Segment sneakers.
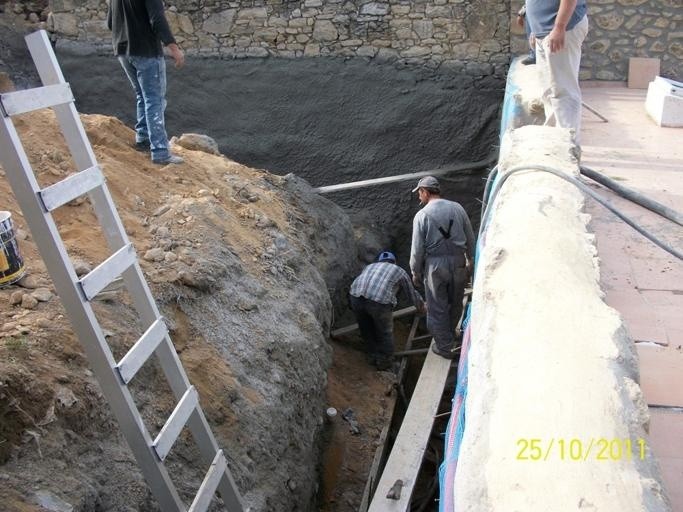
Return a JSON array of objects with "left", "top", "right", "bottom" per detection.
[
  {"left": 522, "top": 58, "right": 535, "bottom": 64},
  {"left": 369, "top": 359, "right": 392, "bottom": 370},
  {"left": 136, "top": 140, "right": 183, "bottom": 163},
  {"left": 432, "top": 344, "right": 453, "bottom": 358}
]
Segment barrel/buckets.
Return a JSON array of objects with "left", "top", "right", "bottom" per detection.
[{"left": 0, "top": 210, "right": 27, "bottom": 289}]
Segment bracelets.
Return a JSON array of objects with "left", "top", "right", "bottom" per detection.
[{"left": 517, "top": 12, "right": 525, "bottom": 18}]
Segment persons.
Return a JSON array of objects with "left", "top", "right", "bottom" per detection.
[
  {"left": 516, "top": 0, "right": 536, "bottom": 67},
  {"left": 349, "top": 250, "right": 427, "bottom": 372},
  {"left": 106, "top": 0, "right": 186, "bottom": 166},
  {"left": 525, "top": 0, "right": 589, "bottom": 145},
  {"left": 407, "top": 176, "right": 477, "bottom": 359}
]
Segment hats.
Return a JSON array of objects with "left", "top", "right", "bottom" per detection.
[
  {"left": 379, "top": 252, "right": 395, "bottom": 262},
  {"left": 412, "top": 176, "right": 440, "bottom": 192}
]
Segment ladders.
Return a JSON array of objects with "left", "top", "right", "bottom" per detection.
[{"left": 0, "top": 28, "right": 247, "bottom": 512}]
